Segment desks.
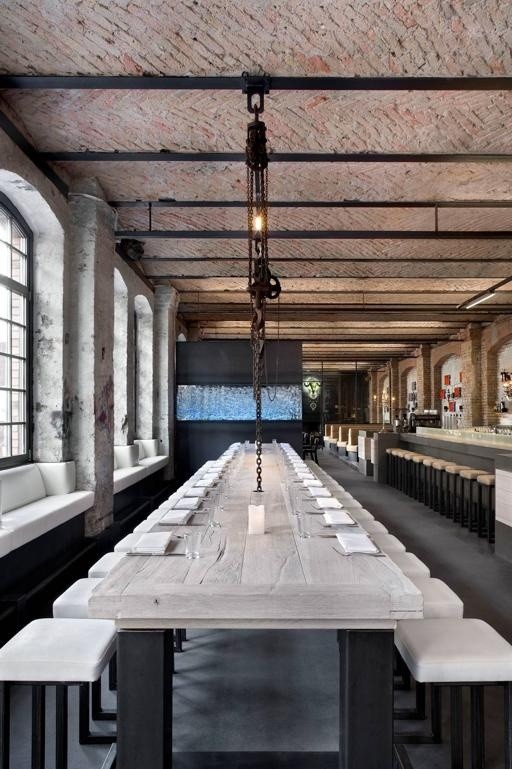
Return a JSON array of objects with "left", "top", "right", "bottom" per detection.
[{"left": 88, "top": 443, "right": 424, "bottom": 769}]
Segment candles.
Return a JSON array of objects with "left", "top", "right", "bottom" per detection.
[{"left": 249, "top": 504, "right": 266, "bottom": 536}]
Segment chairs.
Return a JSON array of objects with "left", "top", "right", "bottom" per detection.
[{"left": 301, "top": 432, "right": 320, "bottom": 466}]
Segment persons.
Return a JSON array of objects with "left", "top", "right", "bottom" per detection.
[{"left": 393, "top": 407, "right": 416, "bottom": 432}]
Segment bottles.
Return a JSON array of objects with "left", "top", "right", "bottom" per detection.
[
  {"left": 494, "top": 397, "right": 505, "bottom": 412},
  {"left": 500, "top": 367, "right": 506, "bottom": 382}
]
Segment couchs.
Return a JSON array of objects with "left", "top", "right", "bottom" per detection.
[{"left": 0, "top": 463, "right": 97, "bottom": 618}]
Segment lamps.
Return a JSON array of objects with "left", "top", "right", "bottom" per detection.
[{"left": 233, "top": 76, "right": 282, "bottom": 492}]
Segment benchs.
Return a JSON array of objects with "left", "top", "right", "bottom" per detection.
[
  {"left": 323, "top": 423, "right": 392, "bottom": 461},
  {"left": 112, "top": 439, "right": 169, "bottom": 515}
]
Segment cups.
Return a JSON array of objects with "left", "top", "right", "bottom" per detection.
[
  {"left": 272, "top": 439, "right": 312, "bottom": 539},
  {"left": 183, "top": 440, "right": 250, "bottom": 560}
]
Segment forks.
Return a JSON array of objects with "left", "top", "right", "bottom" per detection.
[{"left": 332, "top": 546, "right": 386, "bottom": 558}]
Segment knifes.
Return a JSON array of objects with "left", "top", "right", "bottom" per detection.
[
  {"left": 159, "top": 523, "right": 204, "bottom": 527},
  {"left": 320, "top": 534, "right": 372, "bottom": 540}
]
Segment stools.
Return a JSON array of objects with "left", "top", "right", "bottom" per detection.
[
  {"left": 386, "top": 446, "right": 495, "bottom": 546},
  {"left": 392, "top": 617, "right": 510, "bottom": 769},
  {"left": 54, "top": 460, "right": 217, "bottom": 742},
  {"left": 2, "top": 618, "right": 117, "bottom": 769},
  {"left": 303, "top": 460, "right": 464, "bottom": 744}
]
